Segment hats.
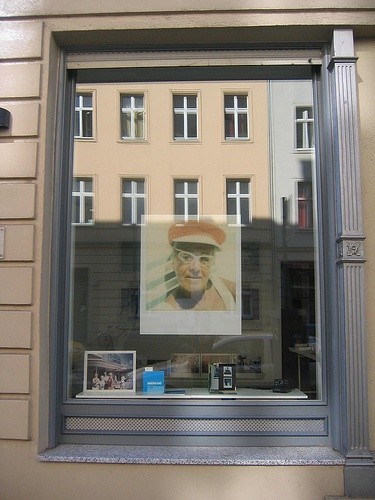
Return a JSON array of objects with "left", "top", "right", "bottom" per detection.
[{"left": 169, "top": 221, "right": 225, "bottom": 250}]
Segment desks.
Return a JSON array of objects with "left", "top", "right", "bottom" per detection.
[{"left": 75, "top": 386, "right": 309, "bottom": 400}]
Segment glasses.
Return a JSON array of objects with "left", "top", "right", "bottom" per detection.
[{"left": 173, "top": 247, "right": 216, "bottom": 268}]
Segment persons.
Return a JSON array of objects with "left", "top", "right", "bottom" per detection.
[
  {"left": 145, "top": 219, "right": 237, "bottom": 310},
  {"left": 91, "top": 371, "right": 127, "bottom": 390}
]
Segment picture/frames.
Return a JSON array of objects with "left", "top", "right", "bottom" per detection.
[
  {"left": 82, "top": 350, "right": 137, "bottom": 394},
  {"left": 219, "top": 363, "right": 237, "bottom": 391}
]
